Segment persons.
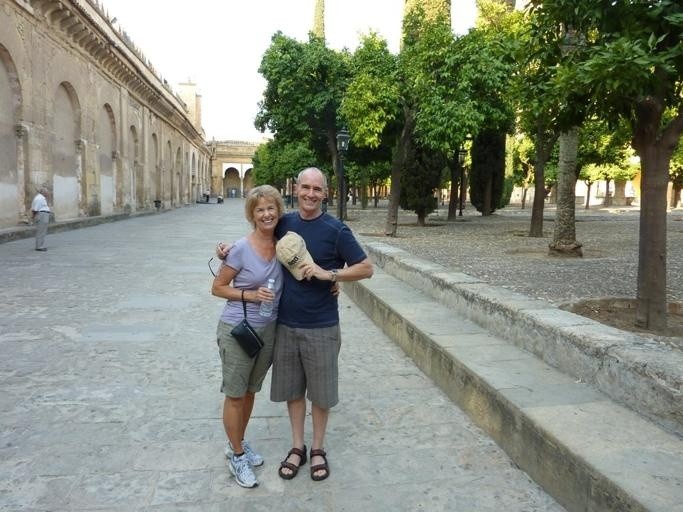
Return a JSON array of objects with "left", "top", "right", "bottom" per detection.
[
  {"left": 215, "top": 167, "right": 373, "bottom": 480},
  {"left": 204, "top": 188, "right": 210, "bottom": 201},
  {"left": 30, "top": 187, "right": 50, "bottom": 251},
  {"left": 210, "top": 185, "right": 341, "bottom": 489}
]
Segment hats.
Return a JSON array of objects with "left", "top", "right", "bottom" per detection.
[{"left": 275, "top": 230, "right": 314, "bottom": 281}]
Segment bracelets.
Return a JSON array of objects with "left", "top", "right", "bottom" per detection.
[{"left": 328, "top": 268, "right": 336, "bottom": 283}]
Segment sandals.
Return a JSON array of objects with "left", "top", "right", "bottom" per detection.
[
  {"left": 278, "top": 445, "right": 307, "bottom": 479},
  {"left": 310, "top": 447, "right": 330, "bottom": 481}
]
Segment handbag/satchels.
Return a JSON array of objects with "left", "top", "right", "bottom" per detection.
[{"left": 230, "top": 289, "right": 264, "bottom": 358}]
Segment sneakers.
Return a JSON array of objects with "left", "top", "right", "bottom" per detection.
[
  {"left": 228, "top": 453, "right": 259, "bottom": 488},
  {"left": 224, "top": 440, "right": 264, "bottom": 466}
]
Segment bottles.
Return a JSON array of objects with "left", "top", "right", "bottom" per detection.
[{"left": 258, "top": 278, "right": 276, "bottom": 317}]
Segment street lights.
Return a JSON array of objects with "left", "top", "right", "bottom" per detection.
[
  {"left": 457, "top": 146, "right": 465, "bottom": 215},
  {"left": 336, "top": 124, "right": 349, "bottom": 222},
  {"left": 372, "top": 175, "right": 376, "bottom": 207}
]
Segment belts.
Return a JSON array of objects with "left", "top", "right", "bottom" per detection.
[{"left": 41, "top": 211, "right": 49, "bottom": 213}]
